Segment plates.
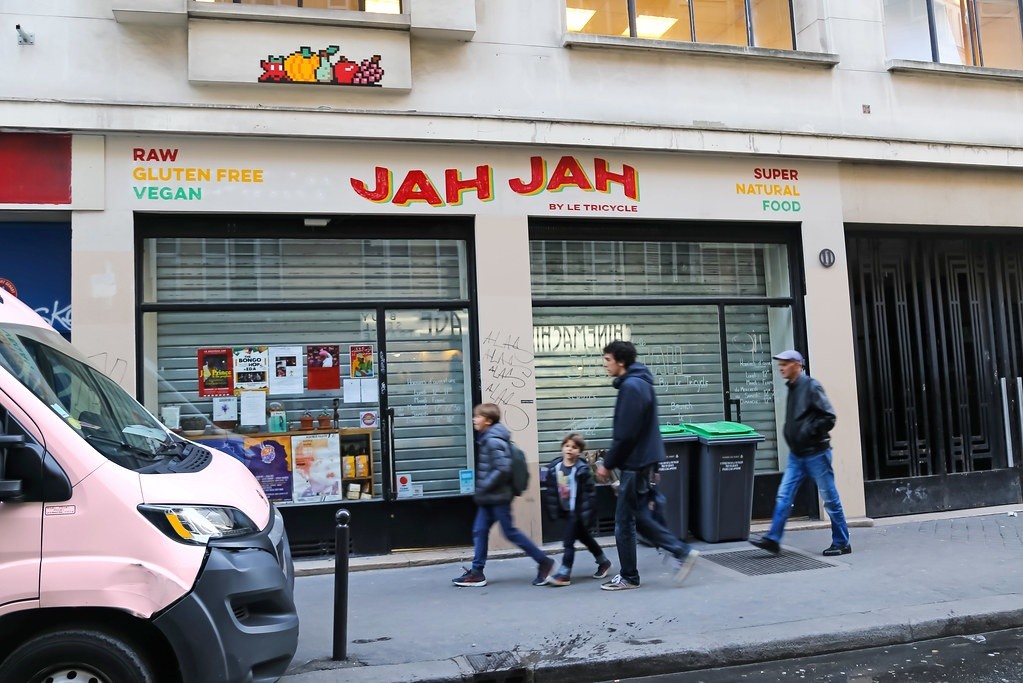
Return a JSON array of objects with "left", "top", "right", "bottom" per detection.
[
  {"left": 211, "top": 428, "right": 233, "bottom": 435},
  {"left": 183, "top": 429, "right": 205, "bottom": 436}
]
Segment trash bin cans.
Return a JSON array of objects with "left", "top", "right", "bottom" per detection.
[
  {"left": 656, "top": 424, "right": 698, "bottom": 541},
  {"left": 680, "top": 419, "right": 765, "bottom": 544}
]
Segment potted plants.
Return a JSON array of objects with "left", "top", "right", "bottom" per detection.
[
  {"left": 317, "top": 409, "right": 331, "bottom": 426},
  {"left": 300, "top": 411, "right": 314, "bottom": 427}
]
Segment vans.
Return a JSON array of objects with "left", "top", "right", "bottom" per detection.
[{"left": 0, "top": 285, "right": 300, "bottom": 683}]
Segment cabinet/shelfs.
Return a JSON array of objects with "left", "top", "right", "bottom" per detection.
[{"left": 184, "top": 427, "right": 376, "bottom": 498}]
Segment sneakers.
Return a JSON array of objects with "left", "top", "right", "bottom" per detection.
[
  {"left": 600, "top": 574, "right": 640, "bottom": 590},
  {"left": 673, "top": 548, "right": 699, "bottom": 583},
  {"left": 452, "top": 566, "right": 487, "bottom": 587},
  {"left": 532, "top": 558, "right": 561, "bottom": 586}
]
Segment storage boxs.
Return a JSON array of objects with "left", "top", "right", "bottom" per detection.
[
  {"left": 347, "top": 484, "right": 375, "bottom": 499},
  {"left": 268, "top": 410, "right": 286, "bottom": 433}
]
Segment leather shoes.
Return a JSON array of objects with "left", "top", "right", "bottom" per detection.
[
  {"left": 748, "top": 537, "right": 780, "bottom": 554},
  {"left": 823, "top": 544, "right": 852, "bottom": 556}
]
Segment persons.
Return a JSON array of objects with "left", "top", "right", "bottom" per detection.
[
  {"left": 546, "top": 432, "right": 612, "bottom": 585},
  {"left": 452, "top": 403, "right": 555, "bottom": 587},
  {"left": 746, "top": 350, "right": 853, "bottom": 557},
  {"left": 600, "top": 340, "right": 700, "bottom": 591},
  {"left": 333, "top": 399, "right": 339, "bottom": 428},
  {"left": 294, "top": 438, "right": 339, "bottom": 497},
  {"left": 309, "top": 350, "right": 333, "bottom": 367}
]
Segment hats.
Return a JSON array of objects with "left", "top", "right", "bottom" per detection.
[{"left": 772, "top": 350, "right": 803, "bottom": 364}]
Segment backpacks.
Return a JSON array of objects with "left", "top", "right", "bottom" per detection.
[{"left": 493, "top": 436, "right": 529, "bottom": 496}]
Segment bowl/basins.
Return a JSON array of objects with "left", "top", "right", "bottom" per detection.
[{"left": 237, "top": 425, "right": 260, "bottom": 433}]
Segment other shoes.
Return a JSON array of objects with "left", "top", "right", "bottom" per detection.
[
  {"left": 593, "top": 560, "right": 613, "bottom": 578},
  {"left": 546, "top": 573, "right": 571, "bottom": 586}
]
